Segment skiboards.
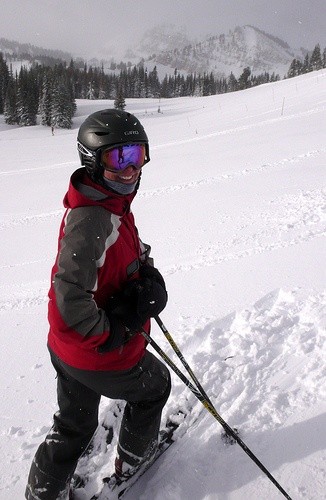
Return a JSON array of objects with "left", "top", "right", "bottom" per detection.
[
  {"left": 90, "top": 356, "right": 238, "bottom": 500},
  {"left": 57, "top": 402, "right": 121, "bottom": 500}
]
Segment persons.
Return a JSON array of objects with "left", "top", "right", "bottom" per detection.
[{"left": 24, "top": 109, "right": 169, "bottom": 500}]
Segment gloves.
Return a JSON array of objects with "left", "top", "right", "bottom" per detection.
[
  {"left": 134, "top": 265, "right": 168, "bottom": 318},
  {"left": 97, "top": 294, "right": 150, "bottom": 354}
]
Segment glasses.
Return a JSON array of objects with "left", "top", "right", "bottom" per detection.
[{"left": 98, "top": 142, "right": 147, "bottom": 171}]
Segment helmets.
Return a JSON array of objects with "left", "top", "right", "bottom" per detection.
[{"left": 77, "top": 108, "right": 150, "bottom": 176}]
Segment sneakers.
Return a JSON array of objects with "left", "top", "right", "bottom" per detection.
[{"left": 116, "top": 435, "right": 158, "bottom": 482}]
